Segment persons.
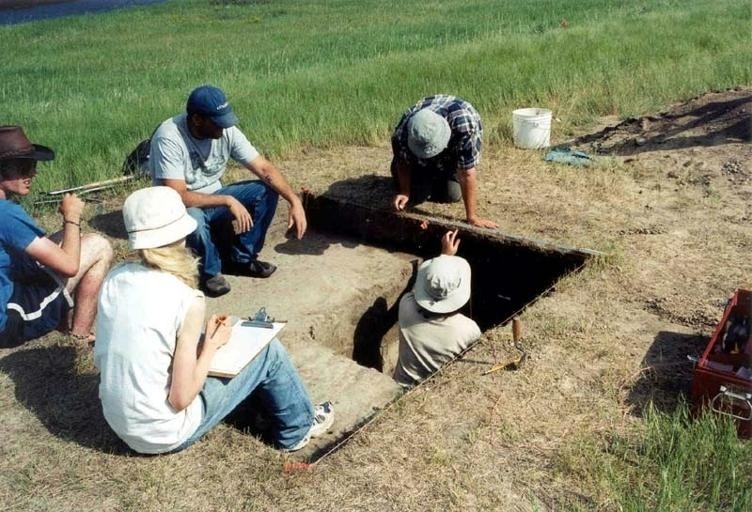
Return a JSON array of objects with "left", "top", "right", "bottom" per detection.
[
  {"left": 92, "top": 187, "right": 334, "bottom": 456},
  {"left": 149, "top": 85, "right": 307, "bottom": 297},
  {"left": 390, "top": 95, "right": 500, "bottom": 229},
  {"left": 0, "top": 125, "right": 113, "bottom": 350},
  {"left": 393, "top": 229, "right": 482, "bottom": 385}
]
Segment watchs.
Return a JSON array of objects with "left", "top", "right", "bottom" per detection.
[{"left": 64, "top": 220, "right": 80, "bottom": 227}]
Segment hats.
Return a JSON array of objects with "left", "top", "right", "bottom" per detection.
[
  {"left": 406, "top": 108, "right": 452, "bottom": 159},
  {"left": 414, "top": 253, "right": 472, "bottom": 313},
  {"left": 186, "top": 85, "right": 239, "bottom": 128},
  {"left": 0, "top": 125, "right": 56, "bottom": 163},
  {"left": 122, "top": 187, "right": 198, "bottom": 252}
]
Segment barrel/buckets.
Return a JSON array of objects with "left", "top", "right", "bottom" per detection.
[{"left": 513, "top": 107, "right": 553, "bottom": 148}]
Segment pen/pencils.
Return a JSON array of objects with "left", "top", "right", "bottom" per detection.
[{"left": 218, "top": 320, "right": 226, "bottom": 327}]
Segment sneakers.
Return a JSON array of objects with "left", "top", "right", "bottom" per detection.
[
  {"left": 234, "top": 260, "right": 277, "bottom": 277},
  {"left": 289, "top": 401, "right": 334, "bottom": 451},
  {"left": 203, "top": 275, "right": 230, "bottom": 296}
]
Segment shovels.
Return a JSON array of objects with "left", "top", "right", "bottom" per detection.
[{"left": 512, "top": 317, "right": 531, "bottom": 370}]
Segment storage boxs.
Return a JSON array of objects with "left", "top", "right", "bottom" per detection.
[{"left": 694, "top": 288, "right": 752, "bottom": 437}]
